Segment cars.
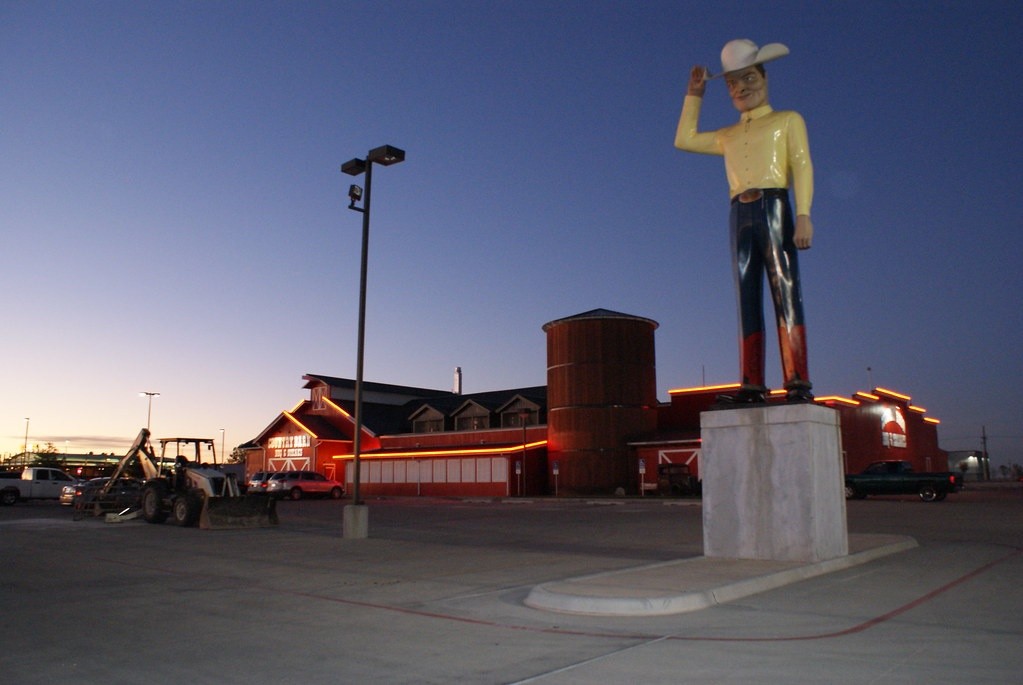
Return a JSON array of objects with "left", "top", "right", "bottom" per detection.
[{"left": 59, "top": 476, "right": 144, "bottom": 511}]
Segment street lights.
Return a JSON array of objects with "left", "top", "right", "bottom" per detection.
[
  {"left": 21, "top": 418, "right": 30, "bottom": 463},
  {"left": 141, "top": 392, "right": 161, "bottom": 452},
  {"left": 339, "top": 145, "right": 407, "bottom": 504}
]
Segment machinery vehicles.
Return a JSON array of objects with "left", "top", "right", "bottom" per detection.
[{"left": 137, "top": 438, "right": 280, "bottom": 532}]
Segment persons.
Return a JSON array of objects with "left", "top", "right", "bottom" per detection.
[{"left": 673, "top": 38, "right": 815, "bottom": 404}]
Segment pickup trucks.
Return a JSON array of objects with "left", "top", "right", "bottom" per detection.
[
  {"left": 0, "top": 466, "right": 80, "bottom": 505},
  {"left": 843, "top": 460, "right": 964, "bottom": 503}
]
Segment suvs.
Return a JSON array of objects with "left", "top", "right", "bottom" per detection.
[{"left": 245, "top": 469, "right": 345, "bottom": 501}]
